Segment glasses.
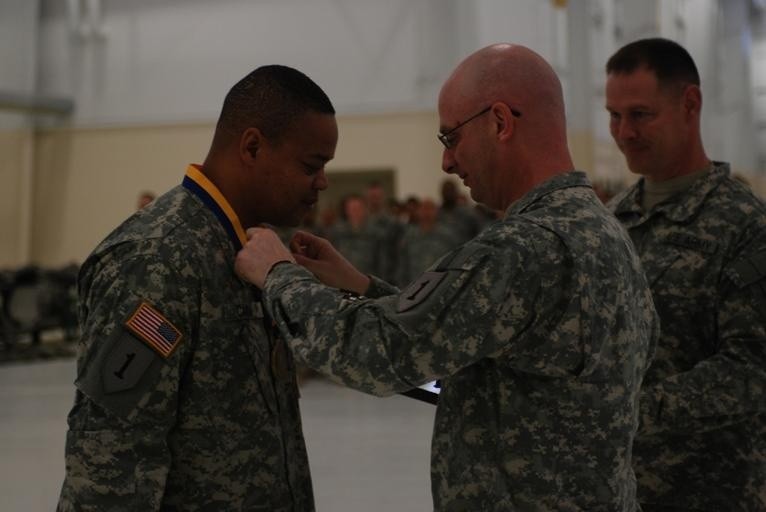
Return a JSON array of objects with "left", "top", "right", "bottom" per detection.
[{"left": 437, "top": 106, "right": 522, "bottom": 149}]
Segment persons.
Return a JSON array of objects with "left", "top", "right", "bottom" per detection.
[
  {"left": 605, "top": 39, "right": 766, "bottom": 512},
  {"left": 56, "top": 65, "right": 339, "bottom": 512},
  {"left": 235, "top": 42, "right": 661, "bottom": 512}
]
